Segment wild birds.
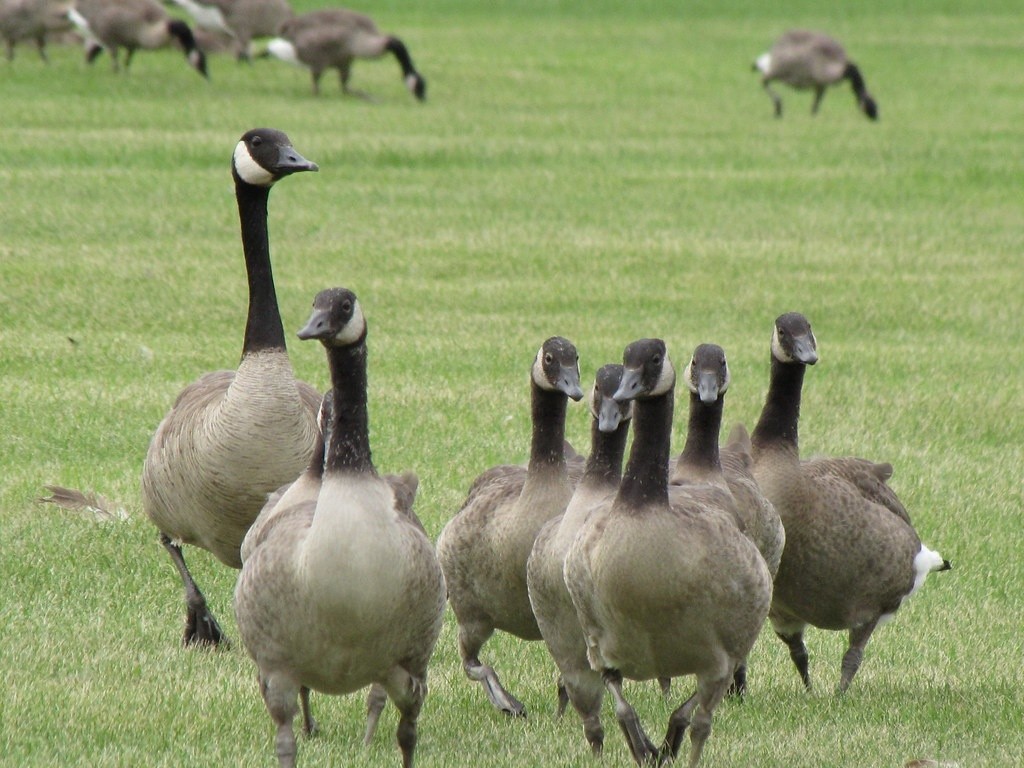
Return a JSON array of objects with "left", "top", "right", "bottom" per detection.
[
  {"left": 141, "top": 127, "right": 324, "bottom": 657},
  {"left": 749, "top": 30, "right": 881, "bottom": 125},
  {"left": 1, "top": 0, "right": 295, "bottom": 85},
  {"left": 228, "top": 287, "right": 447, "bottom": 768},
  {"left": 255, "top": 9, "right": 426, "bottom": 108},
  {"left": 437, "top": 312, "right": 950, "bottom": 768}
]
